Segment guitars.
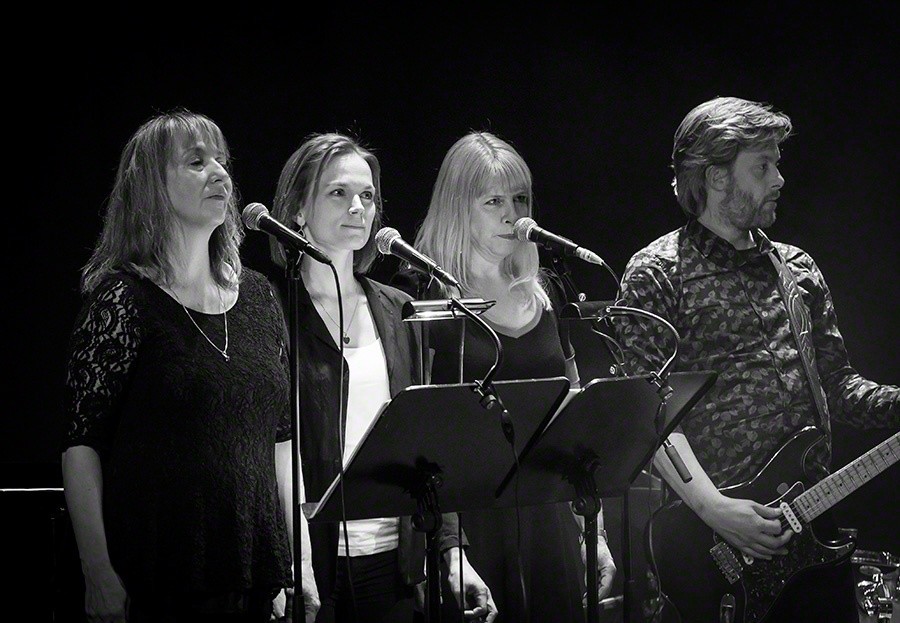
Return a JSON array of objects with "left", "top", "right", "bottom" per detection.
[{"left": 641, "top": 424, "right": 900, "bottom": 623}]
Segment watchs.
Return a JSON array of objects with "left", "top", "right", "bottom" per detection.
[{"left": 579, "top": 530, "right": 608, "bottom": 545}]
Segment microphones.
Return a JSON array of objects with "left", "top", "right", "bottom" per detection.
[
  {"left": 375, "top": 227, "right": 458, "bottom": 287},
  {"left": 513, "top": 217, "right": 604, "bottom": 265},
  {"left": 242, "top": 202, "right": 332, "bottom": 265}
]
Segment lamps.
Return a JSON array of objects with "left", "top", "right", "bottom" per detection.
[
  {"left": 560, "top": 300, "right": 681, "bottom": 380},
  {"left": 401, "top": 298, "right": 502, "bottom": 388}
]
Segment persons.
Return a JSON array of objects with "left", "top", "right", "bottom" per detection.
[
  {"left": 389, "top": 133, "right": 617, "bottom": 623},
  {"left": 60, "top": 112, "right": 321, "bottom": 623},
  {"left": 607, "top": 97, "right": 900, "bottom": 623},
  {"left": 262, "top": 133, "right": 499, "bottom": 623}
]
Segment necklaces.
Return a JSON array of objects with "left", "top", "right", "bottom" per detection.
[
  {"left": 166, "top": 280, "right": 231, "bottom": 363},
  {"left": 309, "top": 290, "right": 360, "bottom": 344}
]
